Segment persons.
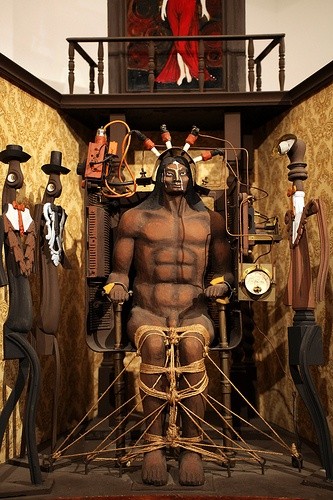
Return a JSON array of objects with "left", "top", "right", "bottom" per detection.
[{"left": 104, "top": 148, "right": 235, "bottom": 487}]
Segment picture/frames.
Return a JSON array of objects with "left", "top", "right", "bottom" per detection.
[{"left": 105, "top": 0, "right": 245, "bottom": 94}]
